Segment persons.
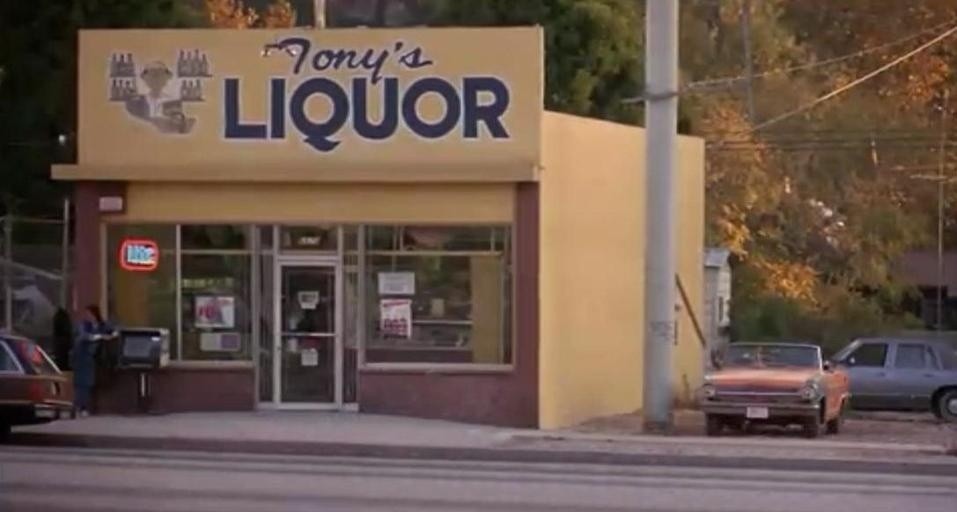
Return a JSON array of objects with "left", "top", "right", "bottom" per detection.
[{"left": 73, "top": 305, "right": 120, "bottom": 418}]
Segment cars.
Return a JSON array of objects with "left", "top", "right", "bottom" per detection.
[
  {"left": 826, "top": 337, "right": 957, "bottom": 421},
  {"left": 700, "top": 339, "right": 848, "bottom": 438},
  {"left": 0, "top": 335, "right": 78, "bottom": 440}
]
